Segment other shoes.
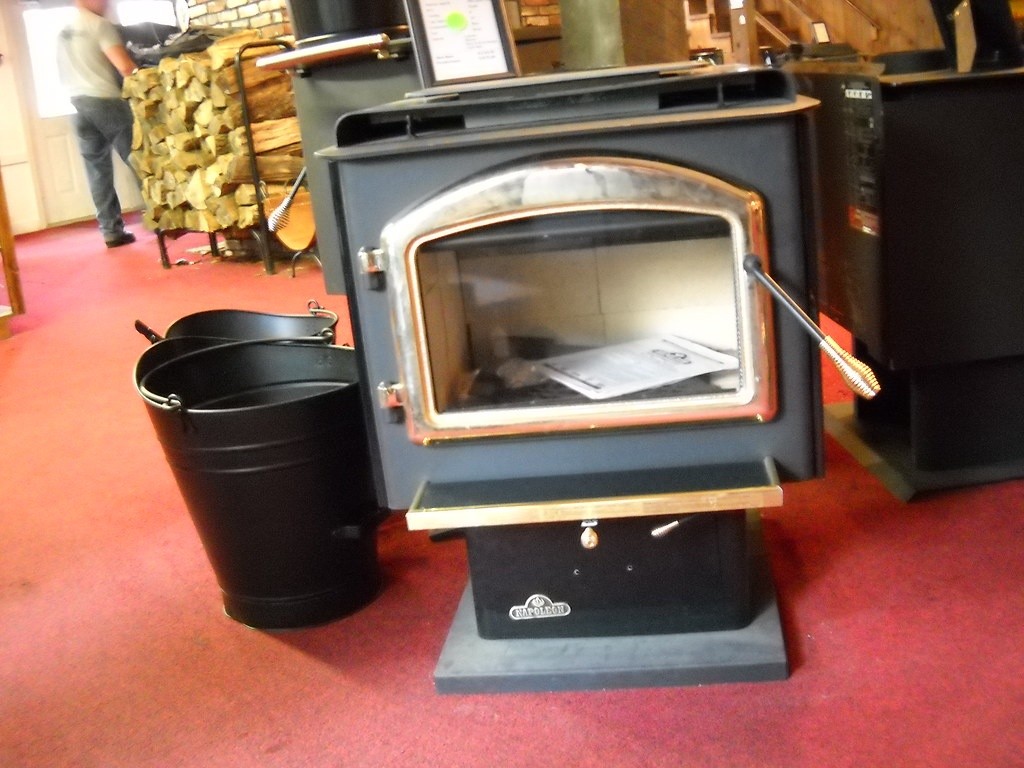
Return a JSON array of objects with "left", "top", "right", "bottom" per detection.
[{"left": 106, "top": 230, "right": 135, "bottom": 248}]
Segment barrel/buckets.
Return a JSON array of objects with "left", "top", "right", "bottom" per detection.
[
  {"left": 164, "top": 299, "right": 338, "bottom": 346},
  {"left": 133, "top": 337, "right": 379, "bottom": 627}
]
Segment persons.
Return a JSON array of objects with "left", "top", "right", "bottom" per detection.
[{"left": 57, "top": 0, "right": 144, "bottom": 248}]
